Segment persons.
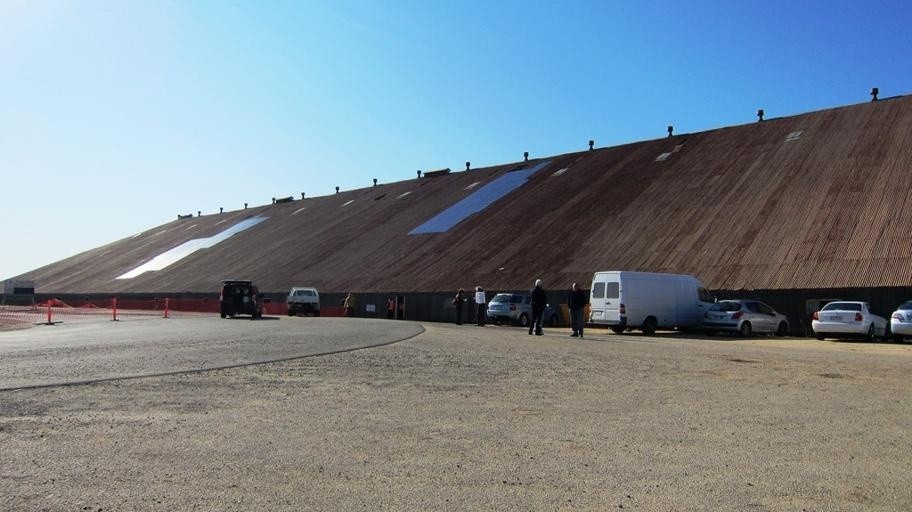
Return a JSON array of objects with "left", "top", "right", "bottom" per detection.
[
  {"left": 343, "top": 292, "right": 353, "bottom": 317},
  {"left": 471, "top": 285, "right": 487, "bottom": 326},
  {"left": 385, "top": 295, "right": 395, "bottom": 319},
  {"left": 567, "top": 281, "right": 587, "bottom": 338},
  {"left": 453, "top": 288, "right": 465, "bottom": 325},
  {"left": 529, "top": 278, "right": 547, "bottom": 335}
]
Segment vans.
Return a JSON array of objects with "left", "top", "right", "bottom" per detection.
[{"left": 219, "top": 279, "right": 265, "bottom": 321}]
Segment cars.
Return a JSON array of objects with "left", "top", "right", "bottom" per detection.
[
  {"left": 808, "top": 295, "right": 912, "bottom": 346},
  {"left": 702, "top": 297, "right": 790, "bottom": 339},
  {"left": 486, "top": 293, "right": 559, "bottom": 329}
]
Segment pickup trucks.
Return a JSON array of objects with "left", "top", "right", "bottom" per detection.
[{"left": 285, "top": 286, "right": 322, "bottom": 318}]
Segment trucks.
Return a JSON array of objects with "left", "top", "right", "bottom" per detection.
[{"left": 587, "top": 272, "right": 714, "bottom": 336}]
